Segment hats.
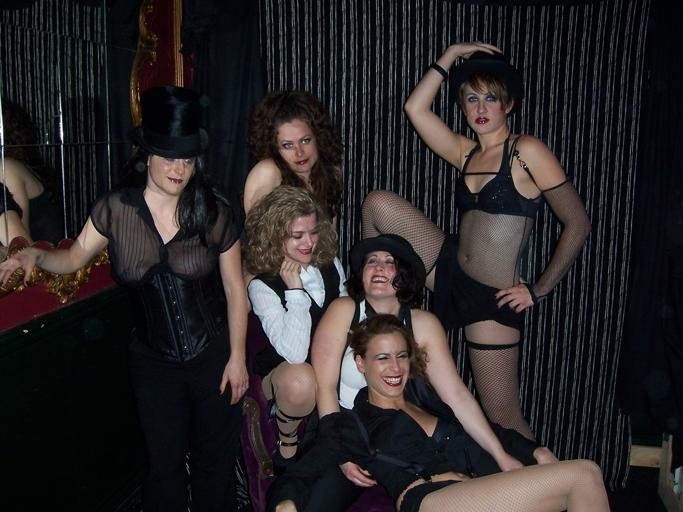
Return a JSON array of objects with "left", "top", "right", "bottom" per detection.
[
  {"left": 348, "top": 233, "right": 427, "bottom": 292},
  {"left": 449, "top": 49, "right": 525, "bottom": 114},
  {"left": 128, "top": 85, "right": 204, "bottom": 159}
]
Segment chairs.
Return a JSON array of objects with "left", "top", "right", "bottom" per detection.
[{"left": 224, "top": 307, "right": 398, "bottom": 510}]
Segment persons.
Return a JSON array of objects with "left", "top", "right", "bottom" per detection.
[
  {"left": 1, "top": 84, "right": 253, "bottom": 510},
  {"left": 241, "top": 38, "right": 611, "bottom": 511}
]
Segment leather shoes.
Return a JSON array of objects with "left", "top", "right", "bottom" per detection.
[{"left": 274, "top": 438, "right": 301, "bottom": 475}]
